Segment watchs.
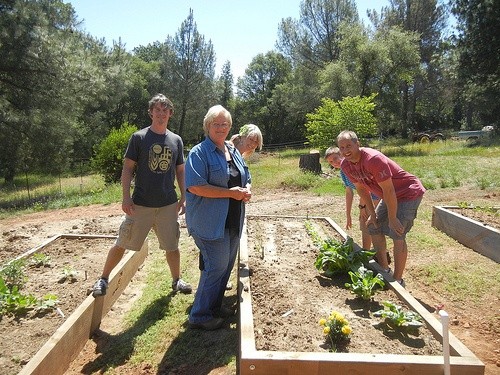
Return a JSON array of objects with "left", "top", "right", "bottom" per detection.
[{"left": 359, "top": 204, "right": 366, "bottom": 209}]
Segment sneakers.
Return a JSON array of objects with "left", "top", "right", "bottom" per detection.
[
  {"left": 92, "top": 276, "right": 108, "bottom": 296},
  {"left": 172, "top": 278, "right": 192, "bottom": 294},
  {"left": 226, "top": 282, "right": 233, "bottom": 290}
]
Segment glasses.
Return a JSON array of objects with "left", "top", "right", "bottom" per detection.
[{"left": 208, "top": 122, "right": 230, "bottom": 129}]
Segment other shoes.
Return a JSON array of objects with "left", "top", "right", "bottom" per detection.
[
  {"left": 189, "top": 317, "right": 225, "bottom": 330},
  {"left": 399, "top": 279, "right": 404, "bottom": 285},
  {"left": 372, "top": 252, "right": 391, "bottom": 265},
  {"left": 215, "top": 306, "right": 234, "bottom": 319},
  {"left": 385, "top": 268, "right": 392, "bottom": 272}
]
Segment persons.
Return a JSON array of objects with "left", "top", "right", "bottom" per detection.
[
  {"left": 336, "top": 130, "right": 426, "bottom": 288},
  {"left": 185, "top": 104, "right": 251, "bottom": 330},
  {"left": 199, "top": 124, "right": 263, "bottom": 290},
  {"left": 324, "top": 146, "right": 391, "bottom": 265},
  {"left": 92, "top": 93, "right": 192, "bottom": 296}
]
